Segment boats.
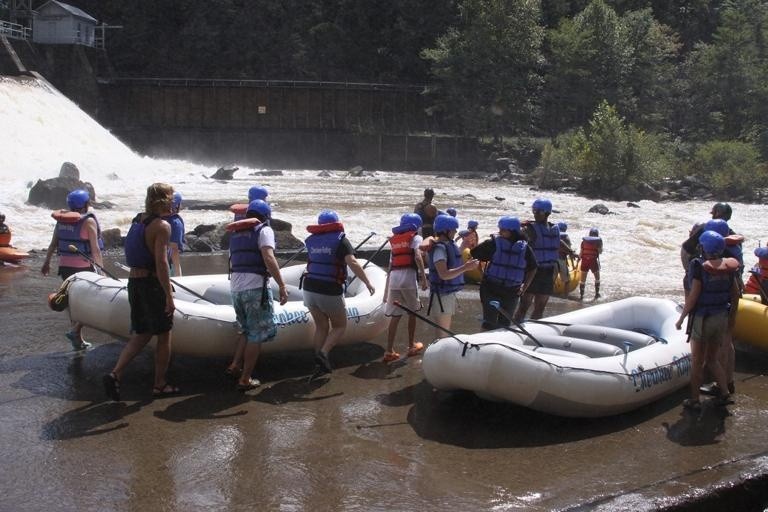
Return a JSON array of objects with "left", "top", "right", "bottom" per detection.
[
  {"left": 60, "top": 258, "right": 390, "bottom": 357},
  {"left": 0, "top": 245, "right": 30, "bottom": 264},
  {"left": 421, "top": 294, "right": 692, "bottom": 418},
  {"left": 731, "top": 292, "right": 768, "bottom": 353},
  {"left": 459, "top": 247, "right": 582, "bottom": 295}
]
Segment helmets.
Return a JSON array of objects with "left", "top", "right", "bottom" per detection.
[
  {"left": 67, "top": 186, "right": 339, "bottom": 224},
  {"left": 400, "top": 188, "right": 599, "bottom": 236},
  {"left": 697, "top": 201, "right": 732, "bottom": 256}
]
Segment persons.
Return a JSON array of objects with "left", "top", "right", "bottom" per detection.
[
  {"left": 161, "top": 191, "right": 185, "bottom": 277},
  {"left": 221, "top": 199, "right": 290, "bottom": 392},
  {"left": 688, "top": 201, "right": 738, "bottom": 238},
  {"left": 229, "top": 185, "right": 273, "bottom": 229},
  {"left": 41, "top": 190, "right": 106, "bottom": 351},
  {"left": 674, "top": 230, "right": 736, "bottom": 411},
  {"left": 299, "top": 209, "right": 376, "bottom": 374},
  {"left": 0, "top": 213, "right": 13, "bottom": 248},
  {"left": 102, "top": 183, "right": 184, "bottom": 401},
  {"left": 411, "top": 189, "right": 604, "bottom": 338},
  {"left": 383, "top": 213, "right": 429, "bottom": 361},
  {"left": 699, "top": 218, "right": 745, "bottom": 397}
]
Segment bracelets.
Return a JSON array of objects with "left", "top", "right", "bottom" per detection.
[{"left": 280, "top": 286, "right": 286, "bottom": 289}]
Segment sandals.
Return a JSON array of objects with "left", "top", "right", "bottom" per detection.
[{"left": 682, "top": 380, "right": 735, "bottom": 409}]
